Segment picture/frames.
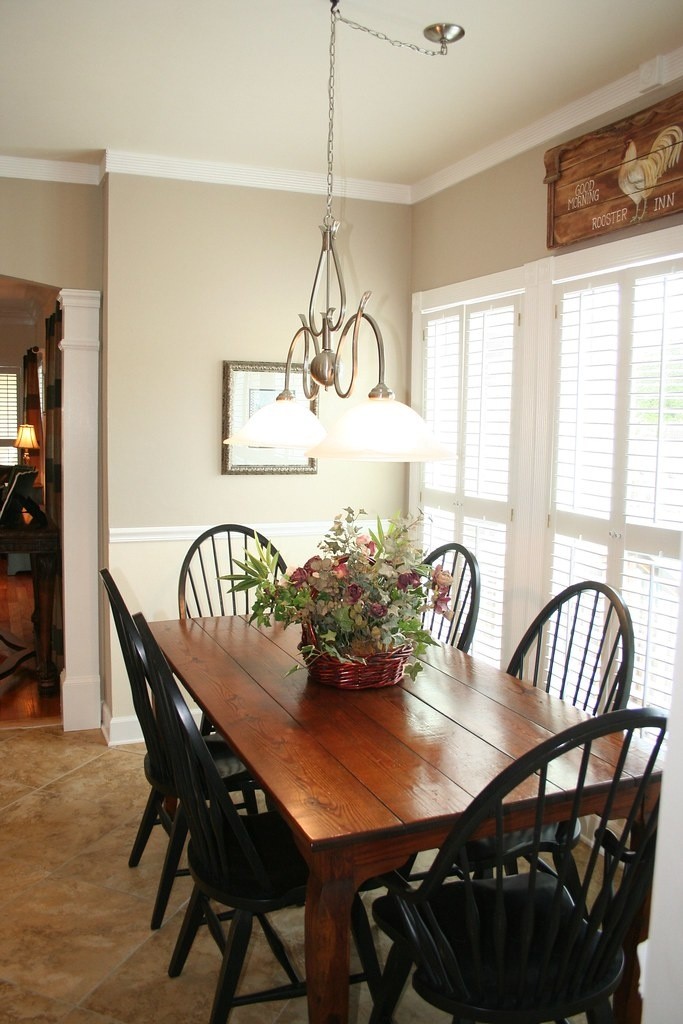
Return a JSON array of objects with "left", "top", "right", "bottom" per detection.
[{"left": 222, "top": 360, "right": 319, "bottom": 475}]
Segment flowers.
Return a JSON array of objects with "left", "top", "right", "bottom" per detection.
[{"left": 216, "top": 504, "right": 453, "bottom": 684}]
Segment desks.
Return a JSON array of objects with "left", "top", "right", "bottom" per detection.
[
  {"left": 0, "top": 506, "right": 60, "bottom": 698},
  {"left": 147, "top": 614, "right": 663, "bottom": 1024}
]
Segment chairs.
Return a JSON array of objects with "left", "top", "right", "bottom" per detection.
[{"left": 101, "top": 524, "right": 667, "bottom": 1024}]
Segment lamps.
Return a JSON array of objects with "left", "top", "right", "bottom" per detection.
[
  {"left": 12, "top": 423, "right": 41, "bottom": 466},
  {"left": 222, "top": 10, "right": 468, "bottom": 461}
]
